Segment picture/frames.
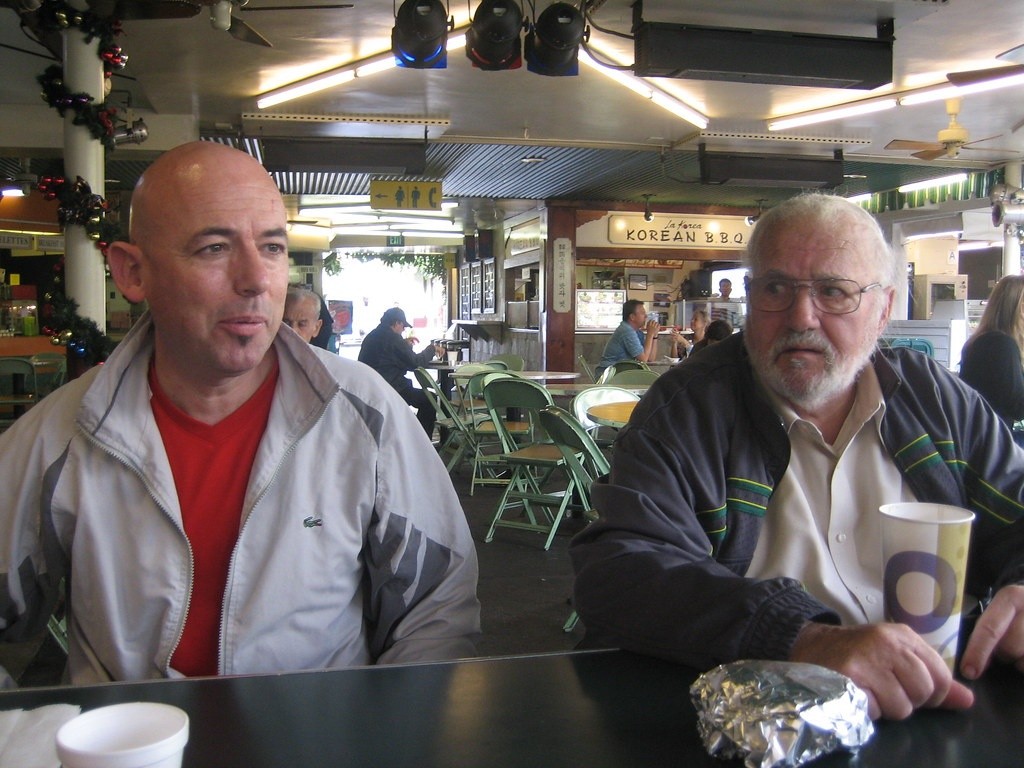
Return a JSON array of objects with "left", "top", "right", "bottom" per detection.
[{"left": 628, "top": 274, "right": 648, "bottom": 290}]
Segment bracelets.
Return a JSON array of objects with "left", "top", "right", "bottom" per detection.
[{"left": 653, "top": 336, "right": 658, "bottom": 339}]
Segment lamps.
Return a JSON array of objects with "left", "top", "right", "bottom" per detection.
[
  {"left": 765, "top": 75, "right": 1024, "bottom": 133},
  {"left": 643, "top": 195, "right": 656, "bottom": 222},
  {"left": 746, "top": 199, "right": 765, "bottom": 225},
  {"left": 255, "top": 0, "right": 710, "bottom": 130}
]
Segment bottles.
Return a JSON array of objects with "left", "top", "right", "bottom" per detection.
[
  {"left": 24, "top": 310, "right": 36, "bottom": 337},
  {"left": 686, "top": 340, "right": 694, "bottom": 358},
  {"left": 652, "top": 313, "right": 659, "bottom": 323}
]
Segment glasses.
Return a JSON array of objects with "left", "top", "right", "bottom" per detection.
[{"left": 747, "top": 276, "right": 881, "bottom": 315}]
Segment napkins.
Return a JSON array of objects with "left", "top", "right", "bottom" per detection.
[{"left": 0, "top": 703, "right": 82, "bottom": 768}]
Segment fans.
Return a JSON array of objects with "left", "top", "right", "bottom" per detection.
[
  {"left": 883, "top": 98, "right": 1020, "bottom": 161},
  {"left": 109, "top": 0, "right": 355, "bottom": 48},
  {"left": 0, "top": 156, "right": 121, "bottom": 197}
]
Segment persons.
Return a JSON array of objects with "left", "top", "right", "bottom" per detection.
[
  {"left": 289, "top": 283, "right": 334, "bottom": 349},
  {"left": 569, "top": 194, "right": 1024, "bottom": 719},
  {"left": 599, "top": 300, "right": 660, "bottom": 365},
  {"left": 1, "top": 140, "right": 483, "bottom": 685},
  {"left": 705, "top": 279, "right": 743, "bottom": 328},
  {"left": 689, "top": 320, "right": 731, "bottom": 356},
  {"left": 358, "top": 308, "right": 445, "bottom": 440},
  {"left": 959, "top": 273, "right": 1024, "bottom": 420},
  {"left": 284, "top": 289, "right": 321, "bottom": 342},
  {"left": 670, "top": 311, "right": 709, "bottom": 358}
]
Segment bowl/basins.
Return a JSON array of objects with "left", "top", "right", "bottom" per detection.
[{"left": 670, "top": 358, "right": 680, "bottom": 363}]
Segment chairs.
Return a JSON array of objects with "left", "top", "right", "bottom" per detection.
[
  {"left": 0, "top": 352, "right": 67, "bottom": 426},
  {"left": 414, "top": 351, "right": 662, "bottom": 550}
]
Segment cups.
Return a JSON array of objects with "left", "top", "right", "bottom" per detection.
[
  {"left": 55, "top": 702, "right": 190, "bottom": 768},
  {"left": 447, "top": 352, "right": 458, "bottom": 368},
  {"left": 878, "top": 502, "right": 976, "bottom": 679}
]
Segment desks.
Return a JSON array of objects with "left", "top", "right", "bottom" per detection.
[
  {"left": 448, "top": 370, "right": 581, "bottom": 487},
  {"left": 539, "top": 381, "right": 653, "bottom": 398},
  {"left": 586, "top": 400, "right": 638, "bottom": 428},
  {"left": 647, "top": 361, "right": 679, "bottom": 369},
  {"left": 0, "top": 631, "right": 1024, "bottom": 768},
  {"left": 419, "top": 363, "right": 461, "bottom": 370}
]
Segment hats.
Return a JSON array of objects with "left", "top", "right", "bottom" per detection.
[{"left": 382, "top": 308, "right": 412, "bottom": 330}]
